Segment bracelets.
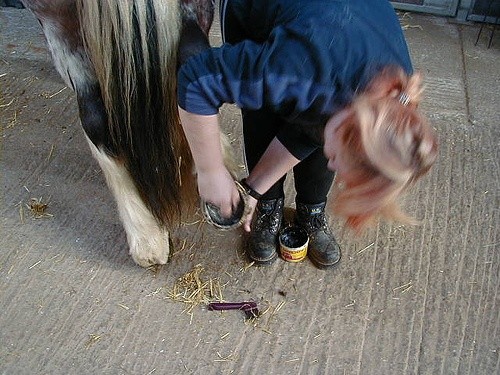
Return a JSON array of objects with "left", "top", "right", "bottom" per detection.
[{"left": 239, "top": 177, "right": 263, "bottom": 202}]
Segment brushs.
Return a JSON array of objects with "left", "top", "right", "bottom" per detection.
[{"left": 209, "top": 302, "right": 259, "bottom": 319}]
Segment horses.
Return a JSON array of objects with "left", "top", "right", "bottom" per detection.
[{"left": 0, "top": 0, "right": 239, "bottom": 269}]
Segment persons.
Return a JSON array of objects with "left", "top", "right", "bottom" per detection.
[{"left": 173, "top": 1, "right": 443, "bottom": 271}]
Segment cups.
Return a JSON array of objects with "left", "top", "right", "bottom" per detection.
[
  {"left": 204, "top": 180, "right": 248, "bottom": 231},
  {"left": 278, "top": 225, "right": 309, "bottom": 263}
]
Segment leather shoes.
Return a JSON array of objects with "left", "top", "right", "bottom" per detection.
[
  {"left": 245, "top": 198, "right": 284, "bottom": 265},
  {"left": 292, "top": 196, "right": 342, "bottom": 270}
]
